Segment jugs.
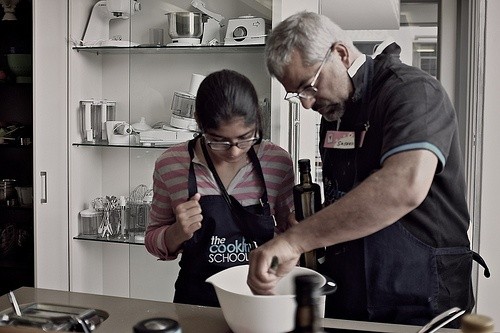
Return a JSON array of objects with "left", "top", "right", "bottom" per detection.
[{"left": 79, "top": 99, "right": 116, "bottom": 144}]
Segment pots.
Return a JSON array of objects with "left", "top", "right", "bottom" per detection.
[{"left": 165, "top": 11, "right": 204, "bottom": 45}]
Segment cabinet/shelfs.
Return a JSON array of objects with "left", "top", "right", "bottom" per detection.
[
  {"left": 0, "top": 0, "right": 33, "bottom": 298},
  {"left": 68, "top": 0, "right": 320, "bottom": 302}
]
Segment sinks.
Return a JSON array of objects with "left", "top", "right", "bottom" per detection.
[{"left": 286, "top": 314, "right": 398, "bottom": 333}]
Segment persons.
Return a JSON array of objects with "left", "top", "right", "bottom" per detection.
[
  {"left": 246, "top": 11, "right": 476, "bottom": 329},
  {"left": 144, "top": 68, "right": 302, "bottom": 309}
]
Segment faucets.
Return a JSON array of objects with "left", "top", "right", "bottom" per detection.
[{"left": 417, "top": 306, "right": 470, "bottom": 332}]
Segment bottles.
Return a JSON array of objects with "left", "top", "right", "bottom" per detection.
[{"left": 293, "top": 159, "right": 327, "bottom": 274}]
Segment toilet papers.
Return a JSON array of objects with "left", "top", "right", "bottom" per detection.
[{"left": 189, "top": 73, "right": 206, "bottom": 96}]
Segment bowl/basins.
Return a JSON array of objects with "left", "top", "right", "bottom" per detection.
[{"left": 205, "top": 264, "right": 326, "bottom": 333}]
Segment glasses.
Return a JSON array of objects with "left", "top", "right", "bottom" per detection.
[
  {"left": 284, "top": 44, "right": 335, "bottom": 104},
  {"left": 202, "top": 119, "right": 260, "bottom": 151}
]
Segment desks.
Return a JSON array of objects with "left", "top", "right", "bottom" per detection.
[{"left": 0, "top": 286, "right": 463, "bottom": 333}]
